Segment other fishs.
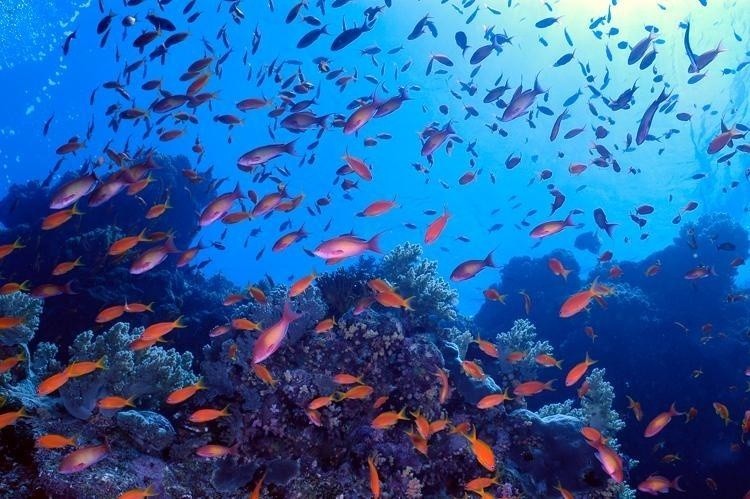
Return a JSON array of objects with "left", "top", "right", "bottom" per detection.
[{"left": 0, "top": 0, "right": 750, "bottom": 499}]
